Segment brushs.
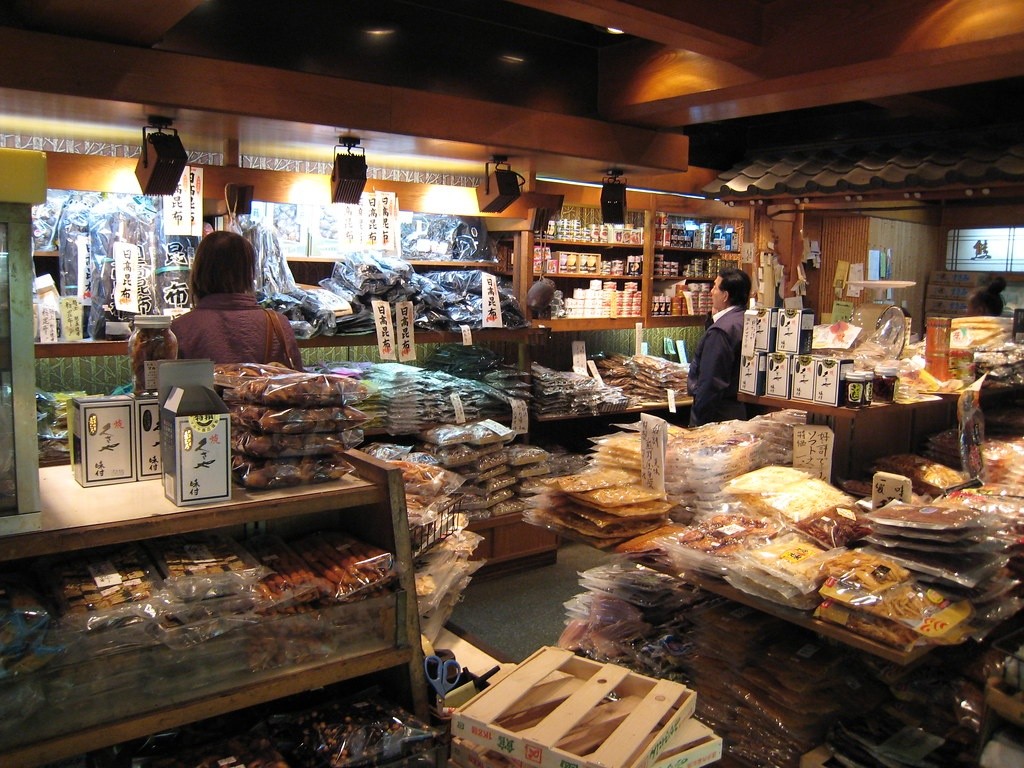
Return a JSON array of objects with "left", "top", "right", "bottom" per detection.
[{"left": 421, "top": 634, "right": 435, "bottom": 659}]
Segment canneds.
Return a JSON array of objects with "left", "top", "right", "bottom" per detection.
[
  {"left": 655, "top": 253, "right": 737, "bottom": 278},
  {"left": 565, "top": 281, "right": 642, "bottom": 318},
  {"left": 875, "top": 368, "right": 899, "bottom": 403},
  {"left": 652, "top": 282, "right": 714, "bottom": 316},
  {"left": 926, "top": 317, "right": 974, "bottom": 387},
  {"left": 857, "top": 371, "right": 874, "bottom": 407},
  {"left": 557, "top": 218, "right": 607, "bottom": 243},
  {"left": 845, "top": 374, "right": 865, "bottom": 408},
  {"left": 128, "top": 315, "right": 178, "bottom": 398}
]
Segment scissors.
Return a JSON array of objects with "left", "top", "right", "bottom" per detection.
[{"left": 424, "top": 655, "right": 461, "bottom": 713}]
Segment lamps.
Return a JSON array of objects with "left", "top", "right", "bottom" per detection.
[
  {"left": 135, "top": 117, "right": 188, "bottom": 196},
  {"left": 600, "top": 169, "right": 628, "bottom": 225},
  {"left": 475, "top": 155, "right": 526, "bottom": 214},
  {"left": 330, "top": 136, "right": 368, "bottom": 205}
]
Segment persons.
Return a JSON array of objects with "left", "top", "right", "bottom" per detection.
[
  {"left": 169, "top": 230, "right": 302, "bottom": 372},
  {"left": 686, "top": 268, "right": 758, "bottom": 430},
  {"left": 964, "top": 275, "right": 1007, "bottom": 318}
]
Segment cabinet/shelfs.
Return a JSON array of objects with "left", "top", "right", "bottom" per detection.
[{"left": 0, "top": 150, "right": 959, "bottom": 768}]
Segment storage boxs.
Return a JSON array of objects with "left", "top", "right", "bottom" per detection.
[
  {"left": 126, "top": 393, "right": 162, "bottom": 481},
  {"left": 813, "top": 358, "right": 855, "bottom": 407},
  {"left": 926, "top": 299, "right": 970, "bottom": 314},
  {"left": 928, "top": 268, "right": 986, "bottom": 287},
  {"left": 987, "top": 271, "right": 1024, "bottom": 284},
  {"left": 925, "top": 312, "right": 969, "bottom": 328},
  {"left": 776, "top": 309, "right": 814, "bottom": 355},
  {"left": 741, "top": 307, "right": 778, "bottom": 352},
  {"left": 739, "top": 353, "right": 766, "bottom": 397},
  {"left": 158, "top": 361, "right": 232, "bottom": 507},
  {"left": 927, "top": 285, "right": 983, "bottom": 300},
  {"left": 713, "top": 225, "right": 723, "bottom": 240},
  {"left": 792, "top": 355, "right": 825, "bottom": 400},
  {"left": 722, "top": 224, "right": 738, "bottom": 251},
  {"left": 70, "top": 394, "right": 137, "bottom": 488},
  {"left": 765, "top": 354, "right": 793, "bottom": 400}
]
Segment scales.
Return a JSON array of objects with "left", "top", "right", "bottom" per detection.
[{"left": 845, "top": 280, "right": 917, "bottom": 360}]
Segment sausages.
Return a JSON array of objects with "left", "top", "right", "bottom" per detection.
[{"left": 241, "top": 539, "right": 396, "bottom": 674}]
